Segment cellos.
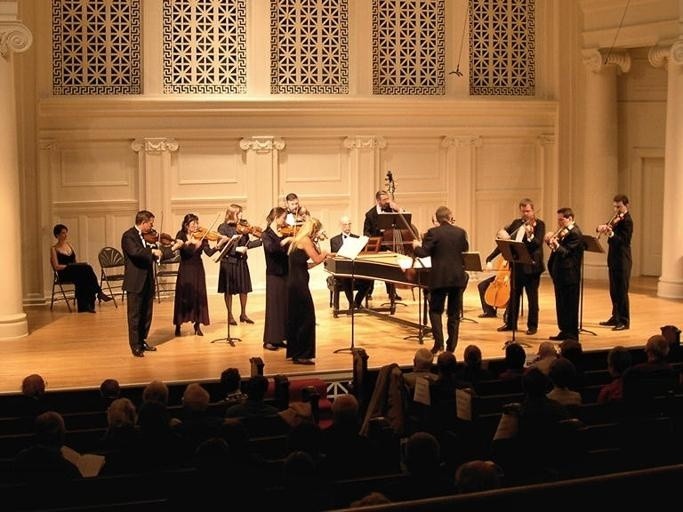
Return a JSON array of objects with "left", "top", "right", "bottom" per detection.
[
  {"left": 483, "top": 255, "right": 511, "bottom": 308},
  {"left": 382, "top": 169, "right": 418, "bottom": 256}
]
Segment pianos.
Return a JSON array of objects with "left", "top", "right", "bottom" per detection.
[{"left": 323, "top": 251, "right": 431, "bottom": 344}]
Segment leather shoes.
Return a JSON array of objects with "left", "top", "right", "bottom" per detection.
[
  {"left": 264, "top": 341, "right": 315, "bottom": 364},
  {"left": 230, "top": 316, "right": 254, "bottom": 325},
  {"left": 133, "top": 342, "right": 155, "bottom": 358},
  {"left": 479, "top": 310, "right": 629, "bottom": 340}
]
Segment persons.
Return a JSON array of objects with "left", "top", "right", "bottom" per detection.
[
  {"left": 476, "top": 240, "right": 522, "bottom": 320},
  {"left": 262, "top": 206, "right": 292, "bottom": 351},
  {"left": 496, "top": 198, "right": 545, "bottom": 334},
  {"left": 364, "top": 189, "right": 404, "bottom": 301},
  {"left": 543, "top": 207, "right": 584, "bottom": 340},
  {"left": 595, "top": 193, "right": 633, "bottom": 331},
  {"left": 172, "top": 214, "right": 229, "bottom": 336},
  {"left": 411, "top": 206, "right": 469, "bottom": 354},
  {"left": 50, "top": 224, "right": 113, "bottom": 313},
  {"left": 217, "top": 203, "right": 262, "bottom": 325},
  {"left": 121, "top": 209, "right": 164, "bottom": 358},
  {"left": 285, "top": 217, "right": 337, "bottom": 364},
  {"left": 1, "top": 324, "right": 682, "bottom": 511},
  {"left": 330, "top": 214, "right": 372, "bottom": 310},
  {"left": 285, "top": 192, "right": 310, "bottom": 238}
]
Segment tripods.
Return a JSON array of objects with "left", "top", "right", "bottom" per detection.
[
  {"left": 502, "top": 262, "right": 532, "bottom": 351},
  {"left": 459, "top": 292, "right": 479, "bottom": 324},
  {"left": 381, "top": 282, "right": 406, "bottom": 310},
  {"left": 334, "top": 261, "right": 369, "bottom": 357},
  {"left": 404, "top": 271, "right": 431, "bottom": 344},
  {"left": 577, "top": 248, "right": 598, "bottom": 337},
  {"left": 211, "top": 255, "right": 241, "bottom": 346}
]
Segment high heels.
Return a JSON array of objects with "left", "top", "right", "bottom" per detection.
[
  {"left": 175, "top": 327, "right": 181, "bottom": 335},
  {"left": 97, "top": 293, "right": 113, "bottom": 302},
  {"left": 194, "top": 324, "right": 204, "bottom": 336}
]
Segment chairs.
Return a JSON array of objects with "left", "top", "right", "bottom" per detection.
[
  {"left": 153, "top": 235, "right": 188, "bottom": 305},
  {"left": 0, "top": 362, "right": 682, "bottom": 511},
  {"left": 97, "top": 247, "right": 129, "bottom": 309},
  {"left": 50, "top": 254, "right": 93, "bottom": 312}
]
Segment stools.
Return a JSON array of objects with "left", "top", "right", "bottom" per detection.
[{"left": 324, "top": 273, "right": 374, "bottom": 319}]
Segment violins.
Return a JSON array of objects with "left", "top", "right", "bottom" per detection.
[
  {"left": 606, "top": 214, "right": 624, "bottom": 234},
  {"left": 295, "top": 207, "right": 310, "bottom": 222},
  {"left": 550, "top": 228, "right": 565, "bottom": 248},
  {"left": 526, "top": 219, "right": 536, "bottom": 238},
  {"left": 235, "top": 220, "right": 262, "bottom": 237},
  {"left": 193, "top": 226, "right": 230, "bottom": 241},
  {"left": 277, "top": 223, "right": 300, "bottom": 236},
  {"left": 142, "top": 228, "right": 176, "bottom": 246}
]
